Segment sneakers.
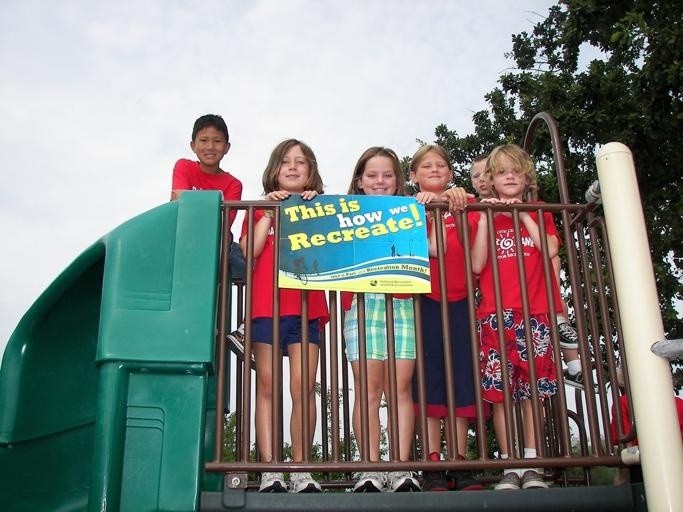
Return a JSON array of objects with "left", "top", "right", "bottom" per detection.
[
  {"left": 353, "top": 471, "right": 384, "bottom": 491},
  {"left": 258, "top": 471, "right": 287, "bottom": 493},
  {"left": 453, "top": 470, "right": 484, "bottom": 490},
  {"left": 289, "top": 471, "right": 321, "bottom": 493},
  {"left": 494, "top": 471, "right": 521, "bottom": 490},
  {"left": 423, "top": 470, "right": 449, "bottom": 490},
  {"left": 522, "top": 470, "right": 549, "bottom": 488},
  {"left": 558, "top": 321, "right": 580, "bottom": 349},
  {"left": 564, "top": 369, "right": 599, "bottom": 393},
  {"left": 225, "top": 330, "right": 255, "bottom": 370},
  {"left": 388, "top": 472, "right": 422, "bottom": 492}
]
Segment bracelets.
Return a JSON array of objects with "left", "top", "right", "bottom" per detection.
[{"left": 264, "top": 209, "right": 276, "bottom": 218}]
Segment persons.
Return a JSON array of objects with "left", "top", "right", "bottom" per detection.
[
  {"left": 293, "top": 256, "right": 305, "bottom": 276},
  {"left": 466, "top": 144, "right": 565, "bottom": 491},
  {"left": 468, "top": 153, "right": 600, "bottom": 394},
  {"left": 237, "top": 138, "right": 332, "bottom": 493},
  {"left": 169, "top": 113, "right": 257, "bottom": 370},
  {"left": 338, "top": 146, "right": 448, "bottom": 493},
  {"left": 609, "top": 360, "right": 683, "bottom": 487},
  {"left": 309, "top": 258, "right": 320, "bottom": 275},
  {"left": 391, "top": 244, "right": 396, "bottom": 257},
  {"left": 407, "top": 144, "right": 492, "bottom": 492}
]
